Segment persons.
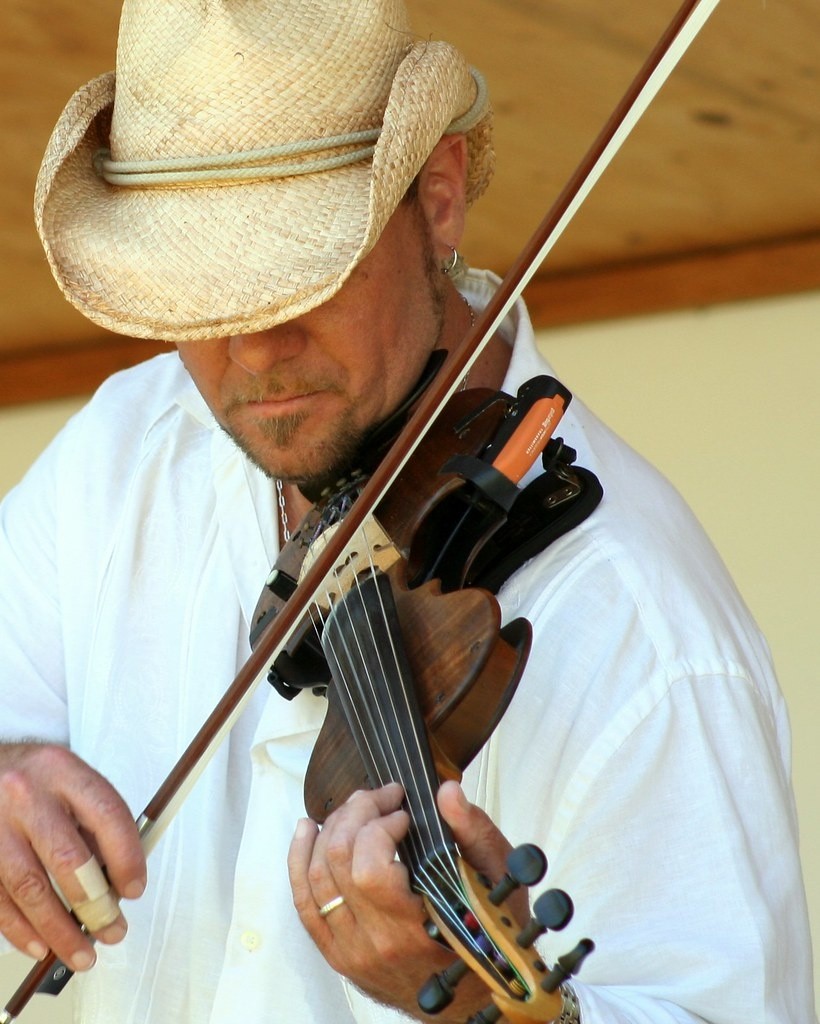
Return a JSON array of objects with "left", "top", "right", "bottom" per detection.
[{"left": 0, "top": 0, "right": 817, "bottom": 1023}]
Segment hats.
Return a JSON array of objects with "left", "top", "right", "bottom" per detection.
[{"left": 33, "top": 0, "right": 495, "bottom": 343}]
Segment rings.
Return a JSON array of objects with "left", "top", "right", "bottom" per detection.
[{"left": 320, "top": 896, "right": 344, "bottom": 918}]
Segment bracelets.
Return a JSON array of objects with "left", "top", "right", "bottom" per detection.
[{"left": 551, "top": 980, "right": 580, "bottom": 1024}]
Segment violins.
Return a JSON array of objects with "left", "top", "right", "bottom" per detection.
[{"left": 247, "top": 385, "right": 598, "bottom": 1024}]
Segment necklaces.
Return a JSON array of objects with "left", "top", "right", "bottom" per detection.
[{"left": 275, "top": 291, "right": 474, "bottom": 541}]
